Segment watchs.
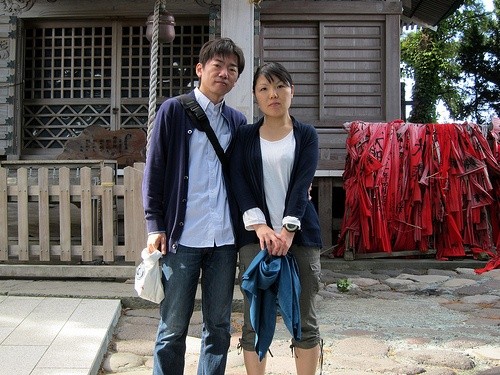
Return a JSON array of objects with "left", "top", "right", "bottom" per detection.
[{"left": 283, "top": 223, "right": 299, "bottom": 232}]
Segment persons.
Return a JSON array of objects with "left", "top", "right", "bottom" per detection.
[
  {"left": 142, "top": 38, "right": 247, "bottom": 375},
  {"left": 223, "top": 62, "right": 324, "bottom": 375}
]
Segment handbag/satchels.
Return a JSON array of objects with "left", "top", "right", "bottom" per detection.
[{"left": 135, "top": 248, "right": 165, "bottom": 304}]
